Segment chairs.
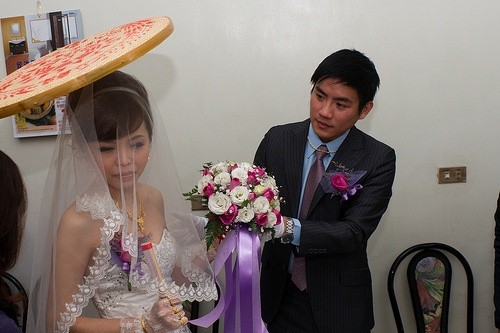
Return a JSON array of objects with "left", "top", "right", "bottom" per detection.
[
  {"left": 388, "top": 243, "right": 475, "bottom": 333},
  {"left": 190, "top": 281, "right": 220, "bottom": 333},
  {"left": 0, "top": 272, "right": 29, "bottom": 333}
]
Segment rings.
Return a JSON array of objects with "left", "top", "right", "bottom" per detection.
[{"left": 168, "top": 299, "right": 186, "bottom": 325}]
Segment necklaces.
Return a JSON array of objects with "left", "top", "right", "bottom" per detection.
[
  {"left": 113, "top": 185, "right": 145, "bottom": 235},
  {"left": 308, "top": 137, "right": 336, "bottom": 154}
]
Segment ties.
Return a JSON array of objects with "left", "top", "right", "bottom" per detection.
[{"left": 290, "top": 145, "right": 328, "bottom": 292}]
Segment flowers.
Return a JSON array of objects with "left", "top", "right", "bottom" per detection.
[
  {"left": 320, "top": 167, "right": 366, "bottom": 201},
  {"left": 183, "top": 159, "right": 287, "bottom": 333},
  {"left": 112, "top": 236, "right": 141, "bottom": 293}
]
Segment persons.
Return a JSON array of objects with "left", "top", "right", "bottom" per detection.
[
  {"left": 0, "top": 150, "right": 30, "bottom": 333},
  {"left": 281, "top": 215, "right": 294, "bottom": 244},
  {"left": 46, "top": 69, "right": 218, "bottom": 333},
  {"left": 254, "top": 50, "right": 396, "bottom": 333}
]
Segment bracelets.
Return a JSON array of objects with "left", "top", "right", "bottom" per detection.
[{"left": 140, "top": 316, "right": 148, "bottom": 333}]
